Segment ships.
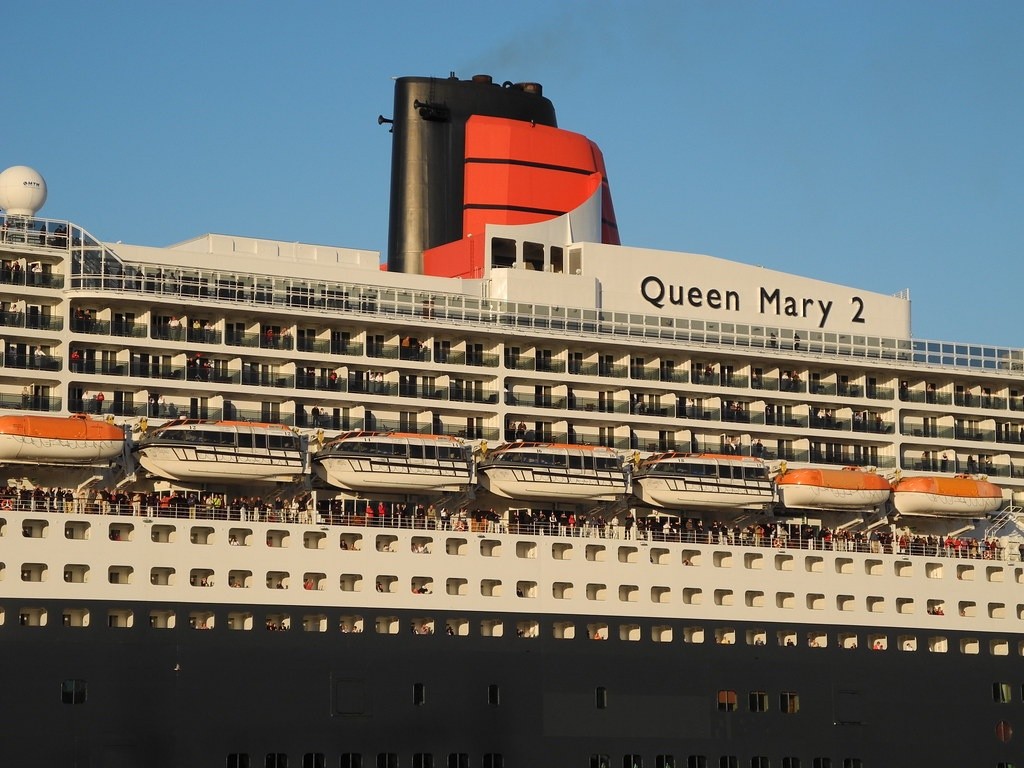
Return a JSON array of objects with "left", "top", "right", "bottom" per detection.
[{"left": 0, "top": 73, "right": 1024, "bottom": 768}]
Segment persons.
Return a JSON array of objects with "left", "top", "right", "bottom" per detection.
[
  {"left": 191, "top": 321, "right": 212, "bottom": 342},
  {"left": 22, "top": 387, "right": 29, "bottom": 409},
  {"left": 32, "top": 265, "right": 41, "bottom": 286},
  {"left": 794, "top": 333, "right": 800, "bottom": 349},
  {"left": 71, "top": 351, "right": 79, "bottom": 372},
  {"left": 34, "top": 345, "right": 46, "bottom": 370},
  {"left": 39, "top": 226, "right": 46, "bottom": 244},
  {"left": 2, "top": 221, "right": 11, "bottom": 239},
  {"left": 158, "top": 395, "right": 178, "bottom": 418},
  {"left": 5, "top": 260, "right": 20, "bottom": 285},
  {"left": 0, "top": 485, "right": 1002, "bottom": 650},
  {"left": 9, "top": 346, "right": 17, "bottom": 368},
  {"left": 187, "top": 359, "right": 1024, "bottom": 479},
  {"left": 770, "top": 333, "right": 775, "bottom": 347},
  {"left": 105, "top": 265, "right": 177, "bottom": 293},
  {"left": 0, "top": 304, "right": 17, "bottom": 326},
  {"left": 401, "top": 336, "right": 427, "bottom": 360},
  {"left": 81, "top": 390, "right": 104, "bottom": 412},
  {"left": 167, "top": 316, "right": 179, "bottom": 341},
  {"left": 72, "top": 305, "right": 91, "bottom": 334},
  {"left": 267, "top": 327, "right": 290, "bottom": 349},
  {"left": 55, "top": 225, "right": 66, "bottom": 245}
]
